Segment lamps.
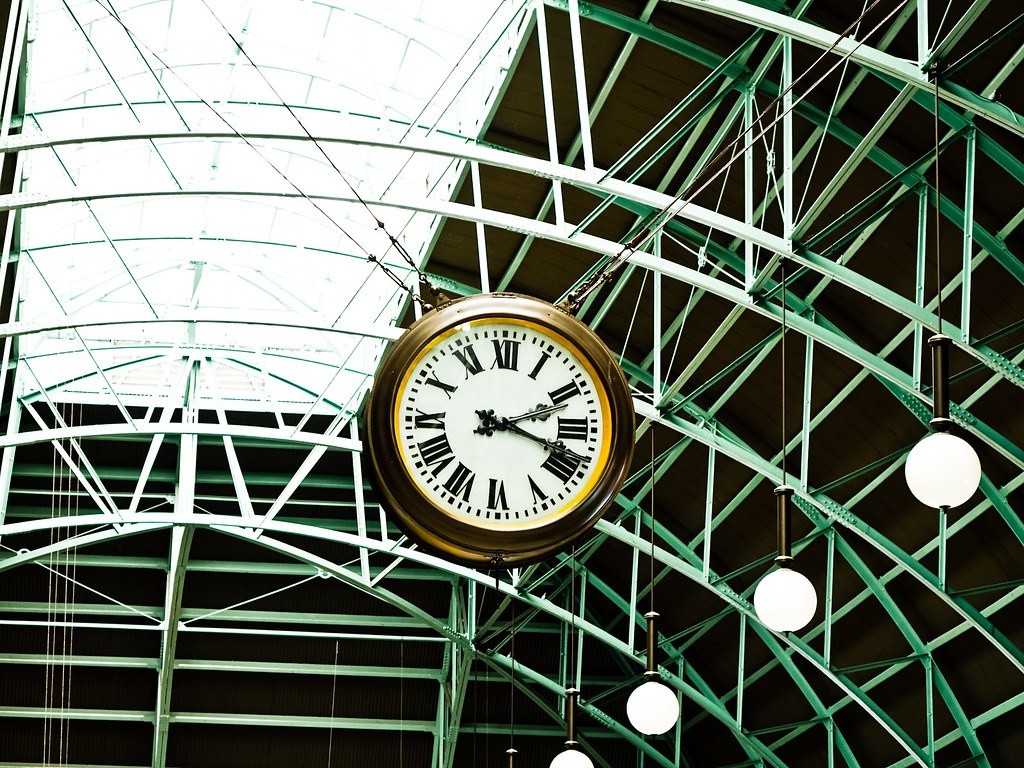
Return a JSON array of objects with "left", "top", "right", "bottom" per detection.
[
  {"left": 627, "top": 420, "right": 679, "bottom": 735},
  {"left": 548, "top": 544, "right": 595, "bottom": 768},
  {"left": 753, "top": 259, "right": 818, "bottom": 641},
  {"left": 904, "top": 69, "right": 980, "bottom": 514}
]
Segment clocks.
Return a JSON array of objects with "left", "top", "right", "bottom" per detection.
[{"left": 361, "top": 291, "right": 637, "bottom": 569}]
